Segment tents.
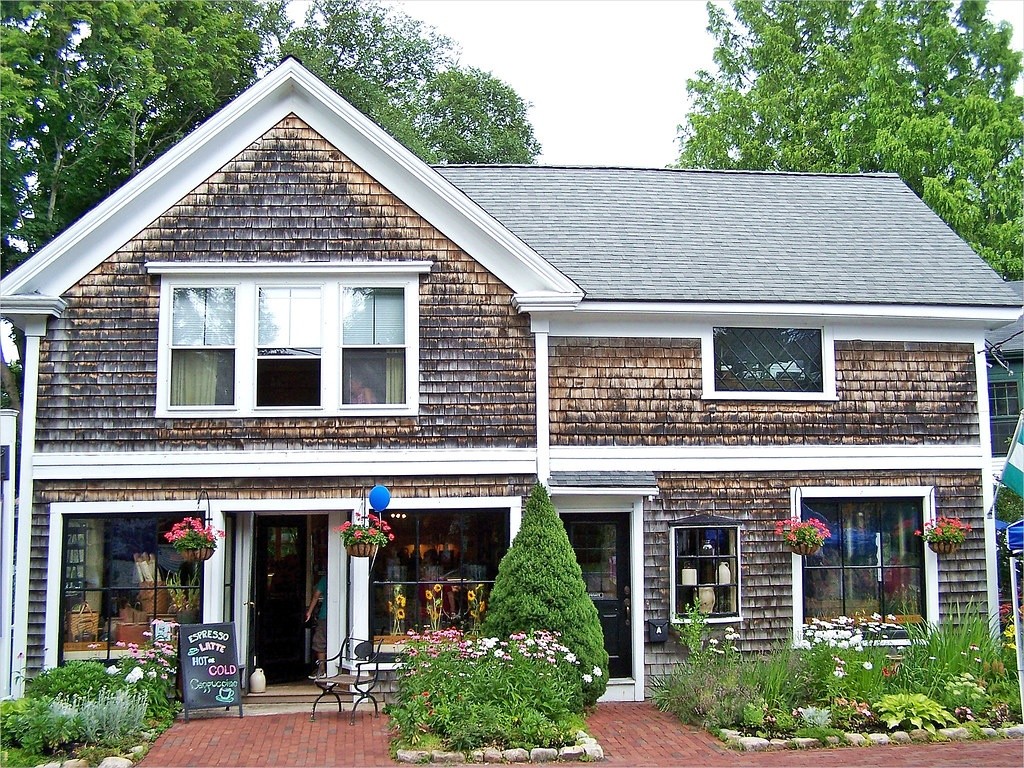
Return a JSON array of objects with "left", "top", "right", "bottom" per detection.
[
  {"left": 1006, "top": 519, "right": 1024, "bottom": 725},
  {"left": 801, "top": 503, "right": 884, "bottom": 615}
]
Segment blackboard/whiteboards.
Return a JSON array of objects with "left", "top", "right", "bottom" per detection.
[{"left": 175, "top": 623, "right": 242, "bottom": 710}]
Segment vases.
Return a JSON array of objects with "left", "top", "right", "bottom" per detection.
[
  {"left": 787, "top": 543, "right": 823, "bottom": 556},
  {"left": 343, "top": 543, "right": 376, "bottom": 556},
  {"left": 928, "top": 543, "right": 962, "bottom": 554},
  {"left": 179, "top": 547, "right": 216, "bottom": 562}
]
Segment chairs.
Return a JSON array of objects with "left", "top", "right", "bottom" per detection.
[{"left": 309, "top": 636, "right": 385, "bottom": 727}]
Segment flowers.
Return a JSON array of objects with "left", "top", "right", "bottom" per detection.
[
  {"left": 773, "top": 515, "right": 831, "bottom": 547},
  {"left": 388, "top": 585, "right": 405, "bottom": 633},
  {"left": 915, "top": 516, "right": 972, "bottom": 543},
  {"left": 465, "top": 582, "right": 485, "bottom": 636},
  {"left": 426, "top": 582, "right": 442, "bottom": 631},
  {"left": 164, "top": 517, "right": 227, "bottom": 552},
  {"left": 333, "top": 513, "right": 394, "bottom": 549}
]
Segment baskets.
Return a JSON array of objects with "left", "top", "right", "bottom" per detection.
[
  {"left": 119, "top": 602, "right": 147, "bottom": 623},
  {"left": 67, "top": 602, "right": 99, "bottom": 642},
  {"left": 139, "top": 581, "right": 168, "bottom": 614}
]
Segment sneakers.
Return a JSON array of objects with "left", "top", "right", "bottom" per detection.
[{"left": 308, "top": 670, "right": 326, "bottom": 679}]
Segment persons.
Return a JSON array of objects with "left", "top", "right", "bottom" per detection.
[
  {"left": 609, "top": 547, "right": 617, "bottom": 599},
  {"left": 398, "top": 548, "right": 507, "bottom": 625},
  {"left": 348, "top": 372, "right": 380, "bottom": 404},
  {"left": 305, "top": 575, "right": 350, "bottom": 680}
]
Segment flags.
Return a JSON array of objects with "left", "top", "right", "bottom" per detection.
[{"left": 999, "top": 409, "right": 1024, "bottom": 499}]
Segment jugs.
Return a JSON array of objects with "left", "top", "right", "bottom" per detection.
[{"left": 75, "top": 619, "right": 97, "bottom": 642}]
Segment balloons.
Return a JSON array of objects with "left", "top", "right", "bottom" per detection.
[{"left": 369, "top": 485, "right": 391, "bottom": 512}]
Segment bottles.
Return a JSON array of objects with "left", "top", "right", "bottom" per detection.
[
  {"left": 715, "top": 562, "right": 730, "bottom": 584},
  {"left": 249, "top": 668, "right": 267, "bottom": 693}
]
